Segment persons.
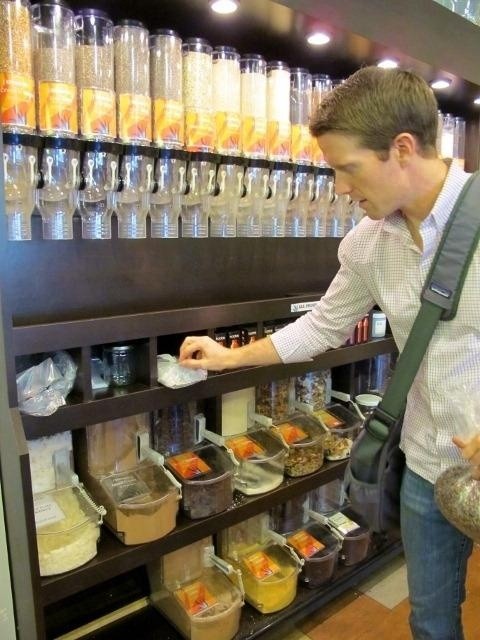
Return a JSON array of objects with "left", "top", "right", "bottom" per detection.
[{"left": 179, "top": 65, "right": 480, "bottom": 640}]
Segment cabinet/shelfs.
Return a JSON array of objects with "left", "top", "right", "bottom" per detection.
[{"left": 0, "top": 0, "right": 480, "bottom": 640}]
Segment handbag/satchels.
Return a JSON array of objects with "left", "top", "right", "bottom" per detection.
[{"left": 343, "top": 411, "right": 406, "bottom": 540}]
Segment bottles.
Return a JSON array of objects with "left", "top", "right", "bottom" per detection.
[
  {"left": 348, "top": 393, "right": 383, "bottom": 424},
  {"left": 342, "top": 307, "right": 386, "bottom": 347},
  {"left": 0, "top": 0, "right": 466, "bottom": 168},
  {"left": 111, "top": 345, "right": 132, "bottom": 387}
]
